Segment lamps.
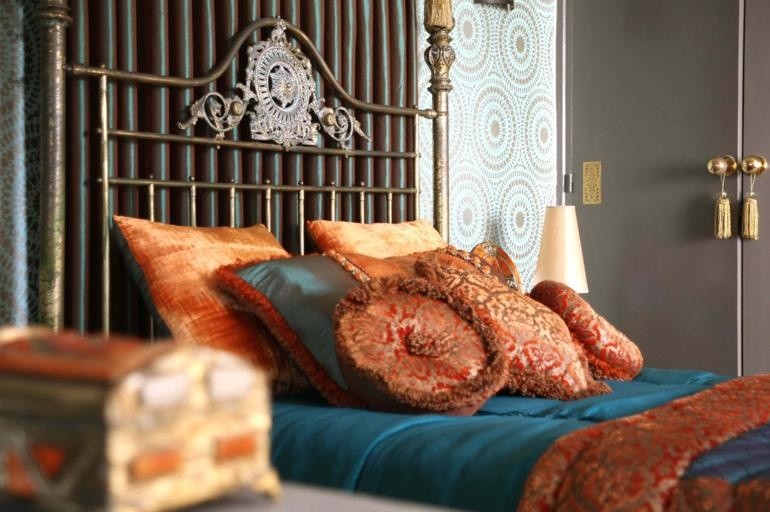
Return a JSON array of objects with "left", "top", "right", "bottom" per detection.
[{"left": 532, "top": 203, "right": 589, "bottom": 295}]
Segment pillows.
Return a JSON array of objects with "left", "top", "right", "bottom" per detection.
[
  {"left": 417, "top": 250, "right": 610, "bottom": 400},
  {"left": 529, "top": 275, "right": 641, "bottom": 384},
  {"left": 334, "top": 277, "right": 506, "bottom": 418},
  {"left": 220, "top": 252, "right": 379, "bottom": 409},
  {"left": 305, "top": 216, "right": 451, "bottom": 256},
  {"left": 113, "top": 214, "right": 310, "bottom": 404}
]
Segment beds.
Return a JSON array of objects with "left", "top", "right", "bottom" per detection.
[{"left": 28, "top": 1, "right": 766, "bottom": 512}]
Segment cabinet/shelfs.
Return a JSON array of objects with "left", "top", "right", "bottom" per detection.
[{"left": 558, "top": 1, "right": 769, "bottom": 387}]
